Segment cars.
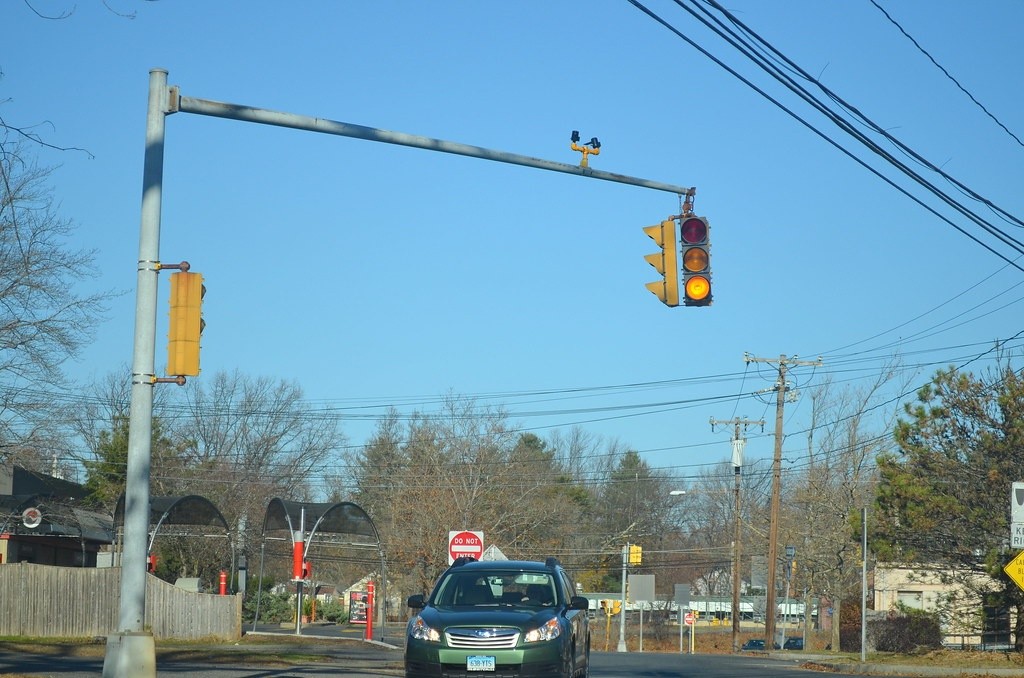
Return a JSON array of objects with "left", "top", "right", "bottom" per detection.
[
  {"left": 780, "top": 636, "right": 805, "bottom": 652},
  {"left": 739, "top": 639, "right": 781, "bottom": 653}
]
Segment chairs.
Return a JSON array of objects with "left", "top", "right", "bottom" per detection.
[
  {"left": 526, "top": 583, "right": 555, "bottom": 604},
  {"left": 460, "top": 585, "right": 493, "bottom": 606}
]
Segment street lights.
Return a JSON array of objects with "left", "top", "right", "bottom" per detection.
[{"left": 668, "top": 488, "right": 742, "bottom": 652}]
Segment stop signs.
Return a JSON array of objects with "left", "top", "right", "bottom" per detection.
[
  {"left": 685, "top": 613, "right": 698, "bottom": 625},
  {"left": 450, "top": 532, "right": 483, "bottom": 565}
]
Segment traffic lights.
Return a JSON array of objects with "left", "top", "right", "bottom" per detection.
[
  {"left": 612, "top": 601, "right": 620, "bottom": 615},
  {"left": 600, "top": 600, "right": 608, "bottom": 616},
  {"left": 679, "top": 216, "right": 715, "bottom": 307},
  {"left": 642, "top": 220, "right": 678, "bottom": 308},
  {"left": 168, "top": 273, "right": 206, "bottom": 377}
]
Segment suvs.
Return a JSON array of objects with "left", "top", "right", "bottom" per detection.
[{"left": 403, "top": 556, "right": 592, "bottom": 678}]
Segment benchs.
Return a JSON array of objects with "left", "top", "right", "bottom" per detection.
[{"left": 492, "top": 593, "right": 526, "bottom": 603}]
